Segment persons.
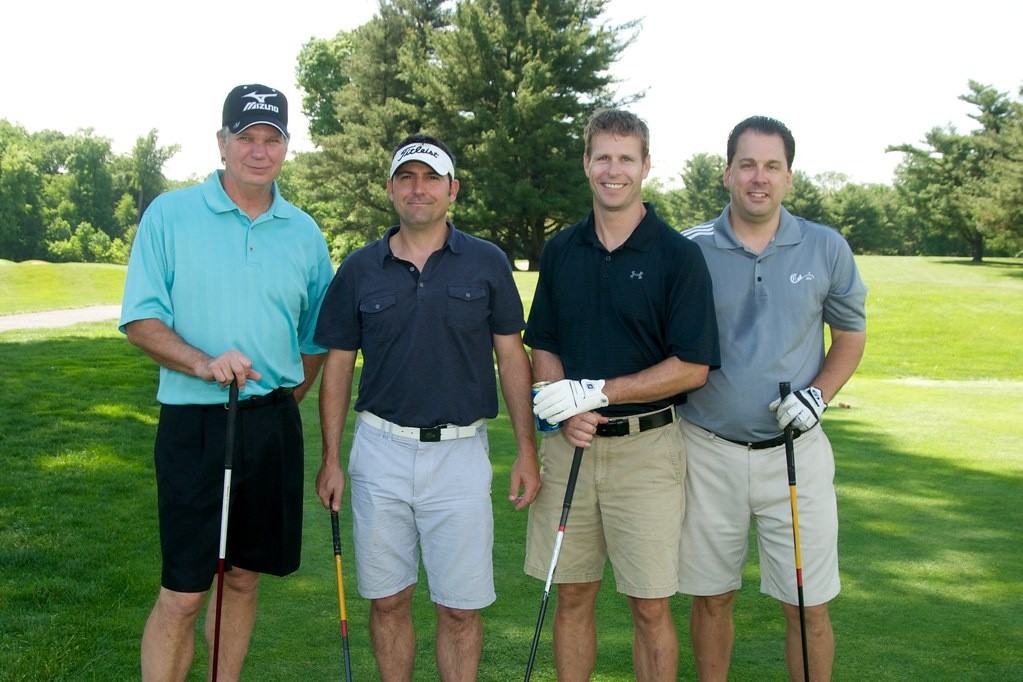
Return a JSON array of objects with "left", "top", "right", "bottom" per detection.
[
  {"left": 678, "top": 114, "right": 872, "bottom": 682},
  {"left": 314, "top": 138, "right": 544, "bottom": 682},
  {"left": 118, "top": 86, "right": 341, "bottom": 682},
  {"left": 526, "top": 110, "right": 721, "bottom": 681}
]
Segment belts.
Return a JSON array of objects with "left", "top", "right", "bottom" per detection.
[
  {"left": 702, "top": 426, "right": 804, "bottom": 450},
  {"left": 585, "top": 407, "right": 674, "bottom": 438},
  {"left": 359, "top": 408, "right": 486, "bottom": 444},
  {"left": 186, "top": 386, "right": 295, "bottom": 412}
]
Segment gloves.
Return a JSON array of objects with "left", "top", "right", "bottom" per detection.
[
  {"left": 533, "top": 376, "right": 609, "bottom": 425},
  {"left": 769, "top": 385, "right": 827, "bottom": 433}
]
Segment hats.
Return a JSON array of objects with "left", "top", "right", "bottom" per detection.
[
  {"left": 221, "top": 83, "right": 290, "bottom": 141},
  {"left": 390, "top": 142, "right": 455, "bottom": 181}
]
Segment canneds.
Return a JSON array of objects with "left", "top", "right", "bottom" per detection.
[{"left": 531, "top": 379, "right": 563, "bottom": 433}]
